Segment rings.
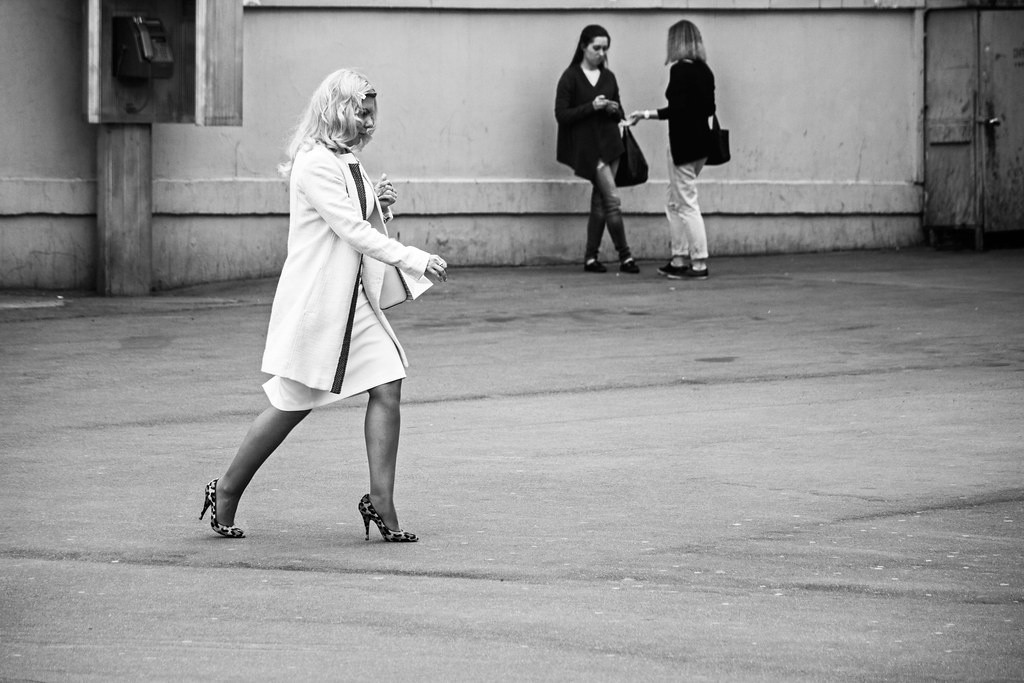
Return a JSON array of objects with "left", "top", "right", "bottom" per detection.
[
  {"left": 439, "top": 262, "right": 442, "bottom": 265},
  {"left": 391, "top": 188, "right": 394, "bottom": 191}
]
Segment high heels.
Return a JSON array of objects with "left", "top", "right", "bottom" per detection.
[
  {"left": 199, "top": 479, "right": 245, "bottom": 539},
  {"left": 359, "top": 493, "right": 418, "bottom": 543}
]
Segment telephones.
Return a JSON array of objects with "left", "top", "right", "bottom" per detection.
[{"left": 113, "top": 13, "right": 173, "bottom": 79}]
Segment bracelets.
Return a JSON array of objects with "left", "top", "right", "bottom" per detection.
[{"left": 643, "top": 110, "right": 650, "bottom": 119}]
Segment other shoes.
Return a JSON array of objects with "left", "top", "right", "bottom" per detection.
[
  {"left": 620, "top": 259, "right": 639, "bottom": 273},
  {"left": 584, "top": 260, "right": 606, "bottom": 272}
]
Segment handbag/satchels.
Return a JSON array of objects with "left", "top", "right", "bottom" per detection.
[
  {"left": 380, "top": 263, "right": 407, "bottom": 310},
  {"left": 704, "top": 114, "right": 731, "bottom": 164},
  {"left": 613, "top": 117, "right": 648, "bottom": 186},
  {"left": 396, "top": 266, "right": 434, "bottom": 302}
]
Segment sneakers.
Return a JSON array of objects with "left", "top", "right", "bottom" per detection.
[
  {"left": 684, "top": 264, "right": 708, "bottom": 279},
  {"left": 657, "top": 263, "right": 687, "bottom": 279}
]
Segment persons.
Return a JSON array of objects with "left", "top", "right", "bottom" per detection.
[
  {"left": 199, "top": 70, "right": 450, "bottom": 543},
  {"left": 626, "top": 19, "right": 716, "bottom": 280},
  {"left": 554, "top": 22, "right": 642, "bottom": 274}
]
